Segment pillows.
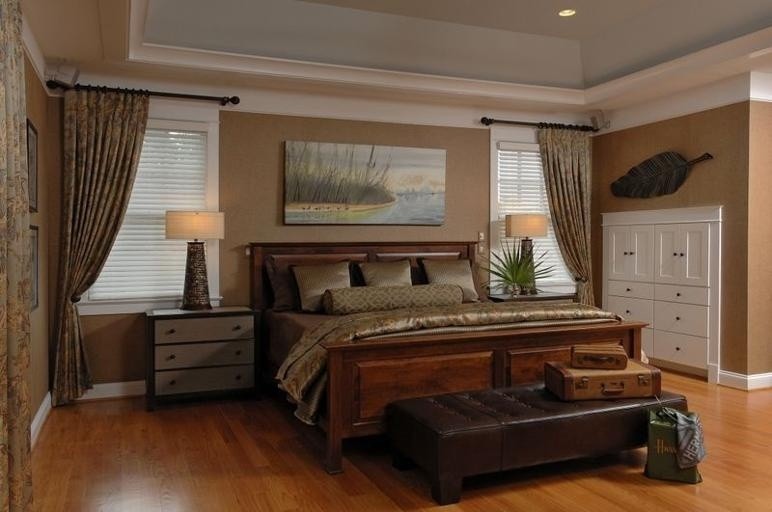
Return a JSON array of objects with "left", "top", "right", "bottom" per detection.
[{"left": 270, "top": 257, "right": 488, "bottom": 316}]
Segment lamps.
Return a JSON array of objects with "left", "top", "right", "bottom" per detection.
[
  {"left": 163, "top": 210, "right": 226, "bottom": 311},
  {"left": 503, "top": 213, "right": 549, "bottom": 295}
]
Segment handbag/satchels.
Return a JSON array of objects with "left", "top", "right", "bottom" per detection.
[{"left": 646, "top": 408, "right": 703, "bottom": 484}]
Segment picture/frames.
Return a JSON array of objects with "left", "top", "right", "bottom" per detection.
[{"left": 27, "top": 118, "right": 40, "bottom": 314}]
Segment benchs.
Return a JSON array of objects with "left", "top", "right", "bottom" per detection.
[{"left": 385, "top": 381, "right": 689, "bottom": 506}]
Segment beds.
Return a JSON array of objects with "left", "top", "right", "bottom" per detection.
[{"left": 247, "top": 238, "right": 651, "bottom": 476}]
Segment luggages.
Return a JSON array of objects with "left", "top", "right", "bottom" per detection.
[
  {"left": 544, "top": 361, "right": 661, "bottom": 402},
  {"left": 571, "top": 344, "right": 628, "bottom": 370}
]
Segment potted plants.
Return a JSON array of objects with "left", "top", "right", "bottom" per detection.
[{"left": 475, "top": 241, "right": 557, "bottom": 299}]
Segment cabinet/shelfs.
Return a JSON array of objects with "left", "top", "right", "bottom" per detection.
[{"left": 600, "top": 206, "right": 724, "bottom": 385}]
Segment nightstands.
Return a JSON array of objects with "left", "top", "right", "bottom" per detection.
[{"left": 144, "top": 304, "right": 262, "bottom": 413}]
